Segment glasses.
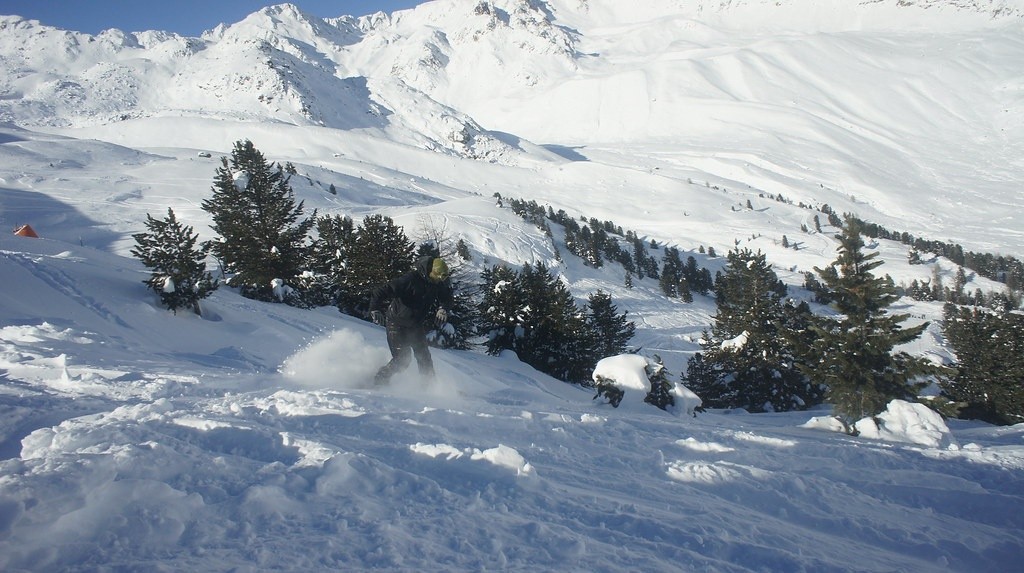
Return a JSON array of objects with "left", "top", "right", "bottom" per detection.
[{"left": 432, "top": 269, "right": 449, "bottom": 281}]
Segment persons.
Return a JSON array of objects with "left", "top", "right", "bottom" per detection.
[{"left": 369, "top": 254, "right": 453, "bottom": 391}]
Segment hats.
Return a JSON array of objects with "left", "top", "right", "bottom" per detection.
[{"left": 429, "top": 258, "right": 449, "bottom": 281}]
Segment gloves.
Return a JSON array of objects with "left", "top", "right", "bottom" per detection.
[
  {"left": 371, "top": 310, "right": 384, "bottom": 326},
  {"left": 434, "top": 309, "right": 447, "bottom": 325}
]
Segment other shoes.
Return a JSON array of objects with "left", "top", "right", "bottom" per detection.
[{"left": 374, "top": 371, "right": 390, "bottom": 386}]
